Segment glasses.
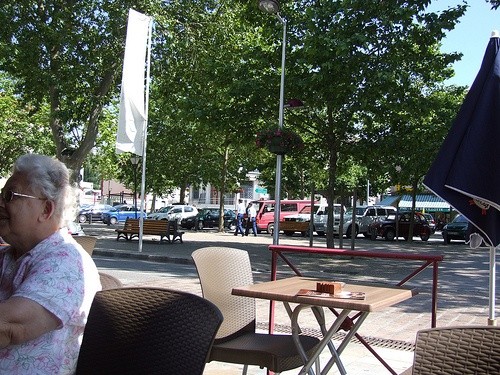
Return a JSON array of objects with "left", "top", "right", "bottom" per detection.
[{"left": 0, "top": 188, "right": 46, "bottom": 201}]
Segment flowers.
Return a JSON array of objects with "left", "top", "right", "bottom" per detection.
[{"left": 255, "top": 125, "right": 304, "bottom": 148}]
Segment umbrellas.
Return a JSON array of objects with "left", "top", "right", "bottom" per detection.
[{"left": 421, "top": 30, "right": 500, "bottom": 319}]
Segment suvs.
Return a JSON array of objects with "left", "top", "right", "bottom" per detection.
[
  {"left": 324, "top": 205, "right": 398, "bottom": 239},
  {"left": 283, "top": 204, "right": 347, "bottom": 236}
]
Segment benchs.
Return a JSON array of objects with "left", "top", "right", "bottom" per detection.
[{"left": 115, "top": 216, "right": 185, "bottom": 243}]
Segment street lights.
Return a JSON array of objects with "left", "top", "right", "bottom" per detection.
[{"left": 257, "top": 0, "right": 288, "bottom": 245}]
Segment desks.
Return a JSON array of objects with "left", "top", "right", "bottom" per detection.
[{"left": 231, "top": 275, "right": 419, "bottom": 375}]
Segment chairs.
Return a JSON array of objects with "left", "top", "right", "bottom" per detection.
[
  {"left": 191, "top": 247, "right": 321, "bottom": 375},
  {"left": 412, "top": 325, "right": 500, "bottom": 375},
  {"left": 74, "top": 288, "right": 225, "bottom": 375}
]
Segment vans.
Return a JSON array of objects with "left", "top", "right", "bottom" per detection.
[{"left": 242, "top": 199, "right": 319, "bottom": 233}]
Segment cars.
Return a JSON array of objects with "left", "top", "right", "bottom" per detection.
[
  {"left": 441, "top": 214, "right": 475, "bottom": 243},
  {"left": 180, "top": 207, "right": 238, "bottom": 231},
  {"left": 102, "top": 203, "right": 147, "bottom": 225},
  {"left": 147, "top": 205, "right": 198, "bottom": 221},
  {"left": 424, "top": 214, "right": 436, "bottom": 232},
  {"left": 76, "top": 204, "right": 114, "bottom": 223},
  {"left": 370, "top": 211, "right": 432, "bottom": 241}
]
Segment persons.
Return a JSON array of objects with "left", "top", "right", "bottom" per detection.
[
  {"left": 235, "top": 199, "right": 257, "bottom": 237},
  {"left": 0, "top": 154, "right": 102, "bottom": 375}
]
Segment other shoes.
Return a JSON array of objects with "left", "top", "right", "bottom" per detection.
[
  {"left": 234, "top": 233, "right": 237, "bottom": 236},
  {"left": 242, "top": 233, "right": 244, "bottom": 237},
  {"left": 245, "top": 233, "right": 248, "bottom": 236},
  {"left": 254, "top": 234, "right": 257, "bottom": 236}
]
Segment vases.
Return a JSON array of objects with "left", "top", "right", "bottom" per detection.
[{"left": 268, "top": 144, "right": 289, "bottom": 155}]
